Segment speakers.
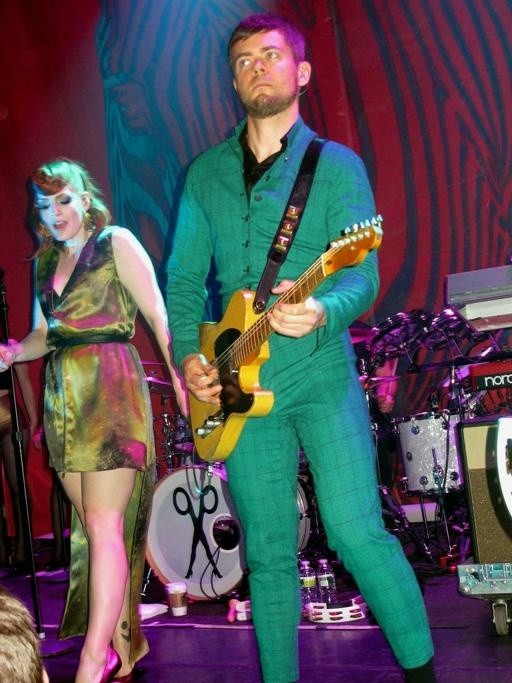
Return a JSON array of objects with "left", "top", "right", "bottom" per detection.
[{"left": 455, "top": 418, "right": 512, "bottom": 565}]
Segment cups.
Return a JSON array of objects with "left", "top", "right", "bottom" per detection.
[{"left": 165, "top": 582, "right": 188, "bottom": 616}]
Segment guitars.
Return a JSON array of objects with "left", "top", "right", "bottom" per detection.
[{"left": 188, "top": 213, "right": 383, "bottom": 462}]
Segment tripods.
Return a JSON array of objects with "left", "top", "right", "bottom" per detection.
[{"left": 362, "top": 347, "right": 465, "bottom": 569}]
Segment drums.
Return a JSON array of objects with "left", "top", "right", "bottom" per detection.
[
  {"left": 145, "top": 464, "right": 312, "bottom": 604},
  {"left": 393, "top": 409, "right": 467, "bottom": 495},
  {"left": 172, "top": 412, "right": 197, "bottom": 451},
  {"left": 371, "top": 309, "right": 468, "bottom": 358}
]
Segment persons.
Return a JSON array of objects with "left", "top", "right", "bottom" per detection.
[
  {"left": 1, "top": 157, "right": 192, "bottom": 682},
  {"left": 1, "top": 592, "right": 54, "bottom": 681},
  {"left": 167, "top": 14, "right": 439, "bottom": 680}
]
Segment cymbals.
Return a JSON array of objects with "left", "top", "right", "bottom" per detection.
[
  {"left": 348, "top": 325, "right": 373, "bottom": 344},
  {"left": 145, "top": 375, "right": 171, "bottom": 388},
  {"left": 139, "top": 360, "right": 167, "bottom": 366},
  {"left": 355, "top": 375, "right": 399, "bottom": 388}
]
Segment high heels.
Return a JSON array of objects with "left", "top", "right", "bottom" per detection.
[{"left": 99, "top": 645, "right": 136, "bottom": 683}]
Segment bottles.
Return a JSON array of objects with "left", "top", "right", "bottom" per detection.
[
  {"left": 316, "top": 559, "right": 337, "bottom": 608},
  {"left": 299, "top": 560, "right": 319, "bottom": 617}
]
